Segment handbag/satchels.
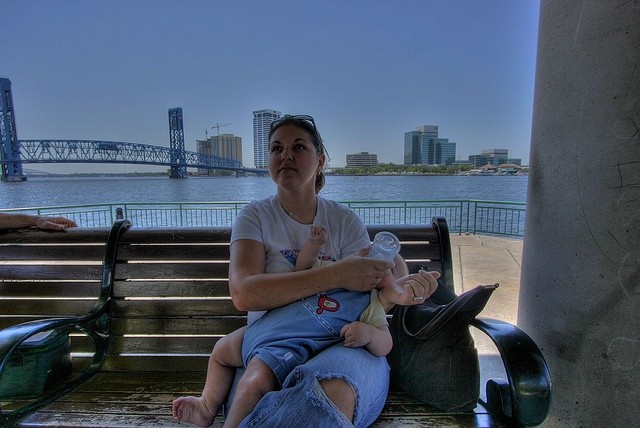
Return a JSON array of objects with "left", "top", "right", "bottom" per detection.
[
  {"left": 0, "top": 317, "right": 73, "bottom": 398},
  {"left": 386, "top": 265, "right": 500, "bottom": 415}
]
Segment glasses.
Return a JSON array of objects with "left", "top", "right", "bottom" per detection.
[{"left": 269, "top": 114, "right": 317, "bottom": 137}]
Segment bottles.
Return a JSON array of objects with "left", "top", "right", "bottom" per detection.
[{"left": 367, "top": 231, "right": 401, "bottom": 262}]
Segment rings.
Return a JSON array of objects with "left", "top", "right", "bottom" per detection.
[{"left": 414, "top": 296, "right": 422, "bottom": 302}]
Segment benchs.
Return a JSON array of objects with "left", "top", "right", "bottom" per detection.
[
  {"left": 0, "top": 219, "right": 131, "bottom": 428},
  {"left": 14, "top": 216, "right": 552, "bottom": 428}
]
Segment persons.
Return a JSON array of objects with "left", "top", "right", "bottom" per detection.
[
  {"left": 229, "top": 116, "right": 441, "bottom": 426},
  {"left": 172, "top": 224, "right": 408, "bottom": 427},
  {"left": 0, "top": 212, "right": 79, "bottom": 231}
]
